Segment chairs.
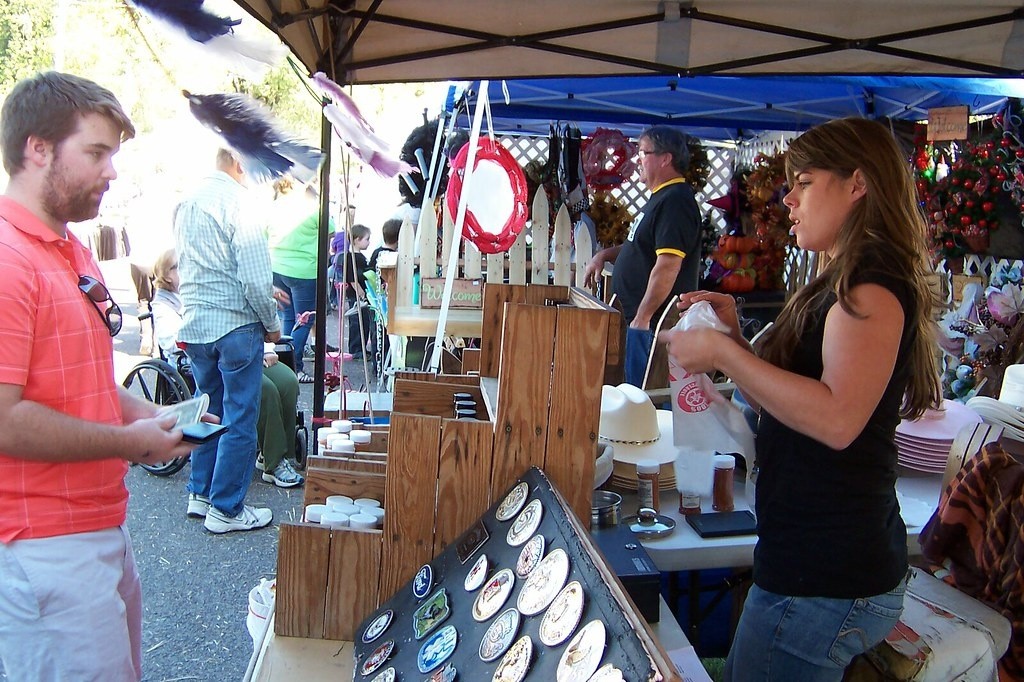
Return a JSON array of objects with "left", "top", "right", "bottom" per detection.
[{"left": 851, "top": 423, "right": 1024, "bottom": 682}]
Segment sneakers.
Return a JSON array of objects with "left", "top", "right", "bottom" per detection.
[
  {"left": 262, "top": 458, "right": 304, "bottom": 487},
  {"left": 204, "top": 504, "right": 272, "bottom": 534},
  {"left": 186, "top": 492, "right": 210, "bottom": 516},
  {"left": 255, "top": 451, "right": 266, "bottom": 471},
  {"left": 302, "top": 346, "right": 315, "bottom": 362}
]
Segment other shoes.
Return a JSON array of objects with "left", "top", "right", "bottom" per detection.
[
  {"left": 353, "top": 352, "right": 372, "bottom": 361},
  {"left": 311, "top": 343, "right": 339, "bottom": 352}
]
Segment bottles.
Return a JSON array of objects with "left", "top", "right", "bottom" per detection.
[
  {"left": 636, "top": 459, "right": 661, "bottom": 516},
  {"left": 711, "top": 455, "right": 736, "bottom": 513},
  {"left": 304, "top": 495, "right": 384, "bottom": 530},
  {"left": 317, "top": 419, "right": 372, "bottom": 453}
]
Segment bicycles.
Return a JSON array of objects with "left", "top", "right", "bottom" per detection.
[{"left": 292, "top": 308, "right": 355, "bottom": 399}]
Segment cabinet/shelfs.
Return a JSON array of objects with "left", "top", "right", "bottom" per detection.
[{"left": 274, "top": 284, "right": 622, "bottom": 640}]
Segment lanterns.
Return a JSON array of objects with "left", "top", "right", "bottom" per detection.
[{"left": 715, "top": 234, "right": 759, "bottom": 292}]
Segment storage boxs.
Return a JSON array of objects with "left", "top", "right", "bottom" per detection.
[
  {"left": 662, "top": 567, "right": 737, "bottom": 652},
  {"left": 590, "top": 522, "right": 661, "bottom": 623}
]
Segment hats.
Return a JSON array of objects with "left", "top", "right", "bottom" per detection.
[
  {"left": 894, "top": 363, "right": 1024, "bottom": 476},
  {"left": 598, "top": 383, "right": 677, "bottom": 490}
]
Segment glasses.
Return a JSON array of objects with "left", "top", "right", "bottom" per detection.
[
  {"left": 637, "top": 151, "right": 663, "bottom": 158},
  {"left": 78, "top": 275, "right": 122, "bottom": 337}
]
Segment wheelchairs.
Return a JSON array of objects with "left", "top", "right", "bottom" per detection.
[{"left": 121, "top": 312, "right": 309, "bottom": 477}]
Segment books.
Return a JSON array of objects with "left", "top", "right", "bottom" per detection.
[{"left": 686, "top": 509, "right": 755, "bottom": 538}]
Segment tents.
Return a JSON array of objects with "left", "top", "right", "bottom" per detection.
[
  {"left": 228, "top": 0, "right": 1024, "bottom": 457},
  {"left": 387, "top": 75, "right": 1024, "bottom": 392}
]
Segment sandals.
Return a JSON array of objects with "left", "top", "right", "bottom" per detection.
[{"left": 298, "top": 374, "right": 314, "bottom": 383}]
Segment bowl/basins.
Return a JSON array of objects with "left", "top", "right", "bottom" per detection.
[{"left": 589, "top": 490, "right": 623, "bottom": 528}]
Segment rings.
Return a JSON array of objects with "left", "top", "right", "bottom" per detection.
[{"left": 279, "top": 293, "right": 281, "bottom": 298}]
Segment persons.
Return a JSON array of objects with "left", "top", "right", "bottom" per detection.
[
  {"left": 584, "top": 128, "right": 709, "bottom": 408},
  {"left": 656, "top": 117, "right": 940, "bottom": 682},
  {"left": 150, "top": 248, "right": 304, "bottom": 486},
  {"left": 260, "top": 170, "right": 403, "bottom": 384},
  {"left": 175, "top": 139, "right": 291, "bottom": 534},
  {"left": 0, "top": 70, "right": 221, "bottom": 682}
]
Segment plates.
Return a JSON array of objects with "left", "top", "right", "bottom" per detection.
[{"left": 893, "top": 397, "right": 984, "bottom": 475}]
